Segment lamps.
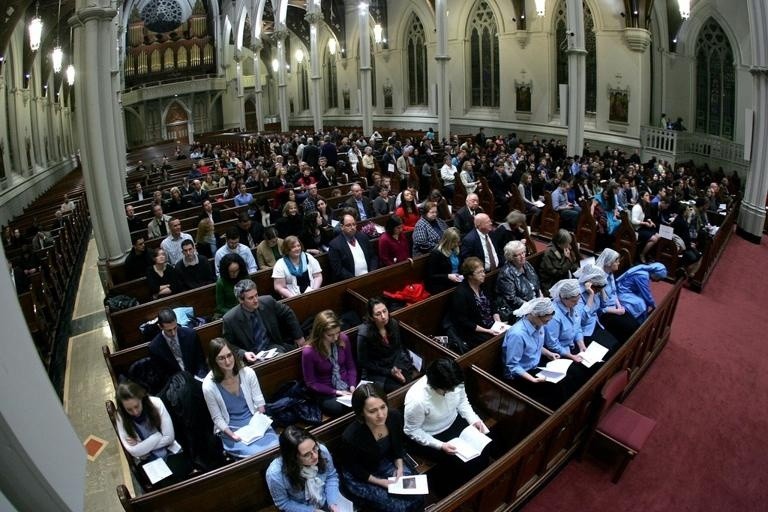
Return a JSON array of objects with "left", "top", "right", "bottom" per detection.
[
  {"left": 269, "top": 0, "right": 691, "bottom": 75},
  {"left": 27, "top": 0, "right": 78, "bottom": 89}
]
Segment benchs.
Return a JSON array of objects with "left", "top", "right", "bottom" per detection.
[{"left": 1, "top": 126, "right": 742, "bottom": 511}]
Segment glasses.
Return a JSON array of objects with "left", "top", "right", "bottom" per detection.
[
  {"left": 299, "top": 443, "right": 321, "bottom": 460},
  {"left": 214, "top": 352, "right": 234, "bottom": 362}
]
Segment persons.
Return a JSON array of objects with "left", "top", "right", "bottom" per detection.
[
  {"left": 612, "top": 92, "right": 628, "bottom": 122},
  {"left": 3, "top": 231, "right": 15, "bottom": 249},
  {"left": 28, "top": 214, "right": 44, "bottom": 234},
  {"left": 8, "top": 263, "right": 30, "bottom": 295},
  {"left": 32, "top": 229, "right": 55, "bottom": 257},
  {"left": 667, "top": 117, "right": 673, "bottom": 129},
  {"left": 671, "top": 117, "right": 687, "bottom": 131},
  {"left": 660, "top": 113, "right": 668, "bottom": 130},
  {"left": 5, "top": 227, "right": 14, "bottom": 236},
  {"left": 11, "top": 228, "right": 29, "bottom": 248},
  {"left": 51, "top": 210, "right": 72, "bottom": 235},
  {"left": 15, "top": 244, "right": 47, "bottom": 273},
  {"left": 61, "top": 194, "right": 76, "bottom": 218}
]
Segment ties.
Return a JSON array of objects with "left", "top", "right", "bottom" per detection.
[
  {"left": 169, "top": 339, "right": 183, "bottom": 359},
  {"left": 349, "top": 238, "right": 356, "bottom": 248},
  {"left": 250, "top": 312, "right": 269, "bottom": 353},
  {"left": 484, "top": 235, "right": 498, "bottom": 273}
]
